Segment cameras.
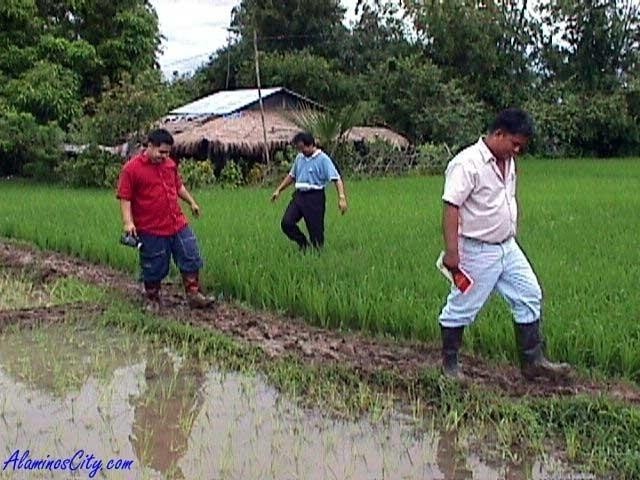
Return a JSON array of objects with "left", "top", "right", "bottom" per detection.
[{"left": 119, "top": 233, "right": 143, "bottom": 249}]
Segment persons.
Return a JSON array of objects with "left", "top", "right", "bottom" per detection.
[
  {"left": 436, "top": 107, "right": 571, "bottom": 383},
  {"left": 270, "top": 132, "right": 348, "bottom": 250},
  {"left": 116, "top": 129, "right": 215, "bottom": 308}
]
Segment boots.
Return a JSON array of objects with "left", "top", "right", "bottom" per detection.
[
  {"left": 180, "top": 269, "right": 216, "bottom": 309},
  {"left": 143, "top": 281, "right": 162, "bottom": 312},
  {"left": 513, "top": 317, "right": 571, "bottom": 380},
  {"left": 439, "top": 321, "right": 465, "bottom": 380}
]
